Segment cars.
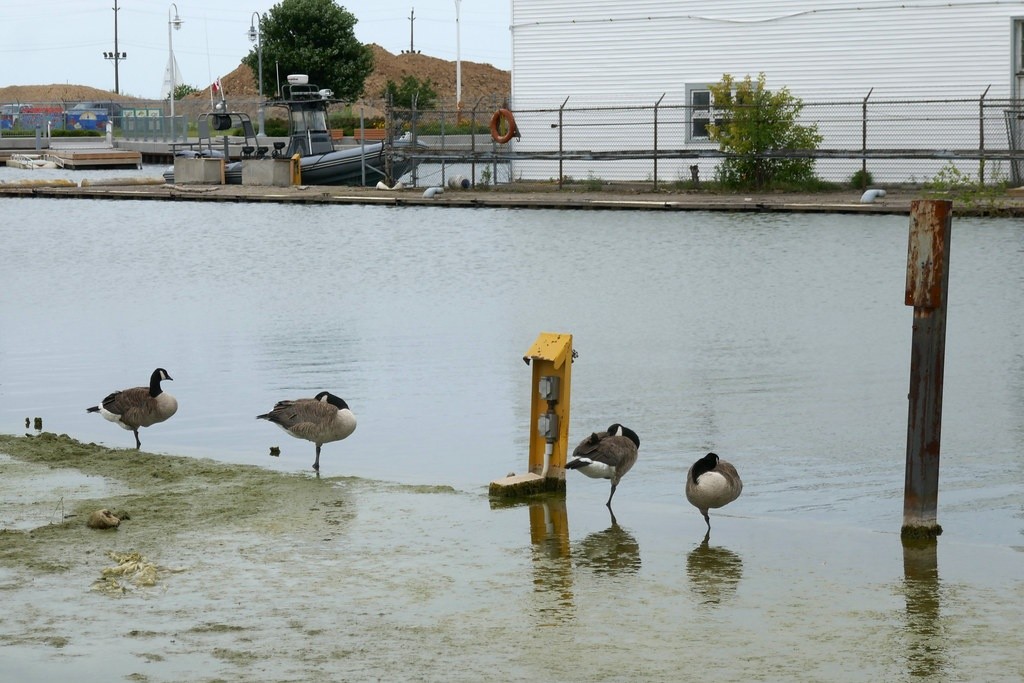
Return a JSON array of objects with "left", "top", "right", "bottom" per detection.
[{"left": 60, "top": 101, "right": 126, "bottom": 128}]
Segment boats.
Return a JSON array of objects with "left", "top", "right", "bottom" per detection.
[{"left": 163, "top": 73, "right": 429, "bottom": 186}]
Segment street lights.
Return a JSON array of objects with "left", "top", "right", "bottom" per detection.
[
  {"left": 249, "top": 11, "right": 268, "bottom": 139},
  {"left": 165, "top": 1, "right": 181, "bottom": 143}
]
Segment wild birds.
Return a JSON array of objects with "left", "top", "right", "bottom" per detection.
[
  {"left": 563, "top": 424, "right": 640, "bottom": 505},
  {"left": 685, "top": 452, "right": 743, "bottom": 528},
  {"left": 85, "top": 368, "right": 178, "bottom": 444},
  {"left": 256, "top": 392, "right": 357, "bottom": 470}
]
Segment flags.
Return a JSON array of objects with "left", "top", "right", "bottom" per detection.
[{"left": 213, "top": 78, "right": 221, "bottom": 92}]
